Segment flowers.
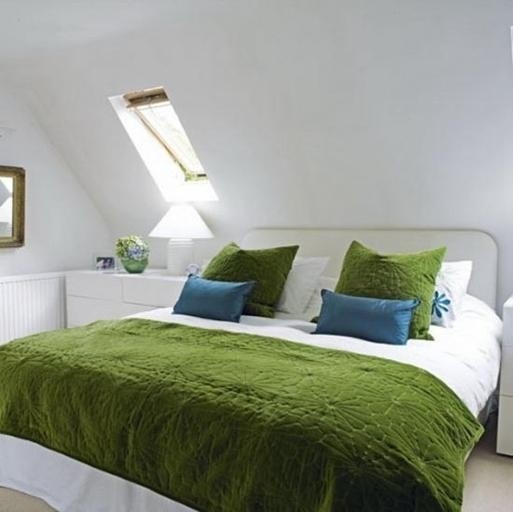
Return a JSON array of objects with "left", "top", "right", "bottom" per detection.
[{"left": 112, "top": 233, "right": 151, "bottom": 263}]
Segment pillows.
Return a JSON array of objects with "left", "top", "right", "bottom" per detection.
[
  {"left": 310, "top": 240, "right": 472, "bottom": 345},
  {"left": 174, "top": 240, "right": 331, "bottom": 325}
]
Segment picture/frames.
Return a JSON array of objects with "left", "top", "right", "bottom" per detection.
[{"left": 0, "top": 165, "right": 27, "bottom": 247}]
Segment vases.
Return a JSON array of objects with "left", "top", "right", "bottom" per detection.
[{"left": 121, "top": 257, "right": 147, "bottom": 274}]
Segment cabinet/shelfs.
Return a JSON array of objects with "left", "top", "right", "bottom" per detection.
[{"left": 62, "top": 266, "right": 186, "bottom": 329}]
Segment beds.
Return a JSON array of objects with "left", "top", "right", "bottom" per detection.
[{"left": 1, "top": 226, "right": 508, "bottom": 512}]
